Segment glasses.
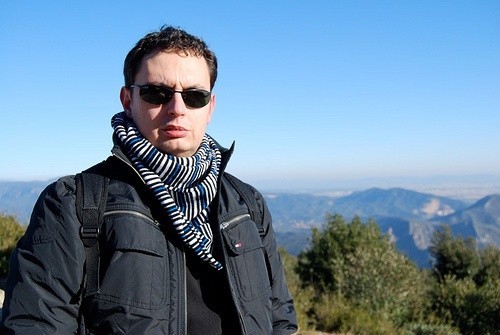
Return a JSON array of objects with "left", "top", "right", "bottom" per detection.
[{"left": 130, "top": 84, "right": 211, "bottom": 107}]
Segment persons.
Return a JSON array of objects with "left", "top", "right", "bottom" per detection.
[{"left": 0, "top": 25, "right": 300, "bottom": 335}]
